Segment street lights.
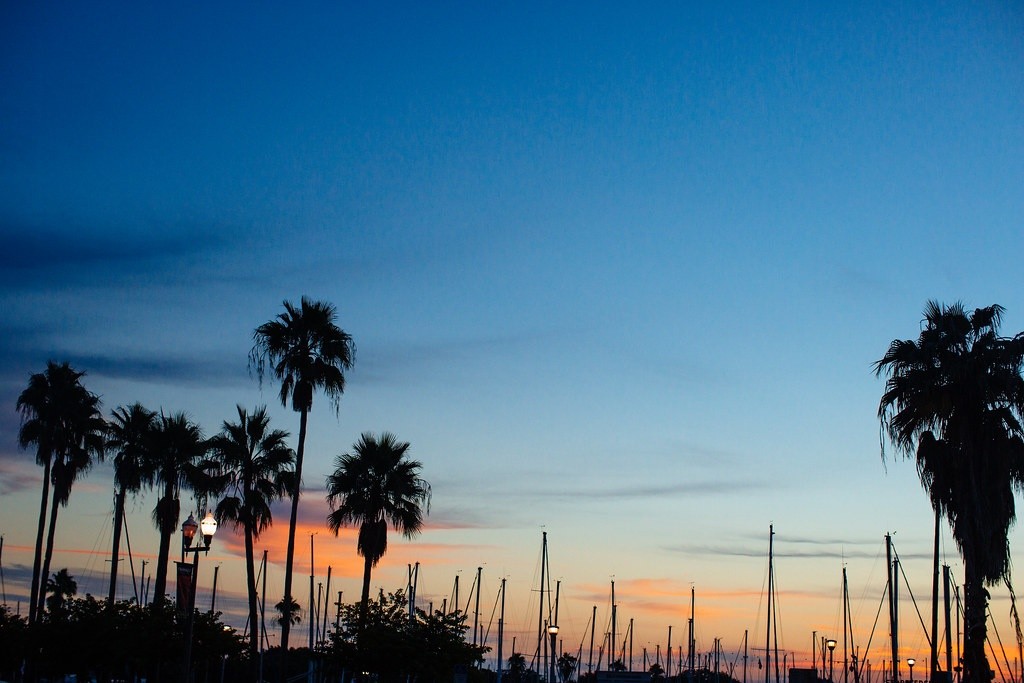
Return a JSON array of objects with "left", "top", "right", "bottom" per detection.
[
  {"left": 548, "top": 625, "right": 559, "bottom": 683},
  {"left": 827, "top": 640, "right": 837, "bottom": 683},
  {"left": 181, "top": 509, "right": 218, "bottom": 683},
  {"left": 907, "top": 659, "right": 916, "bottom": 683}
]
